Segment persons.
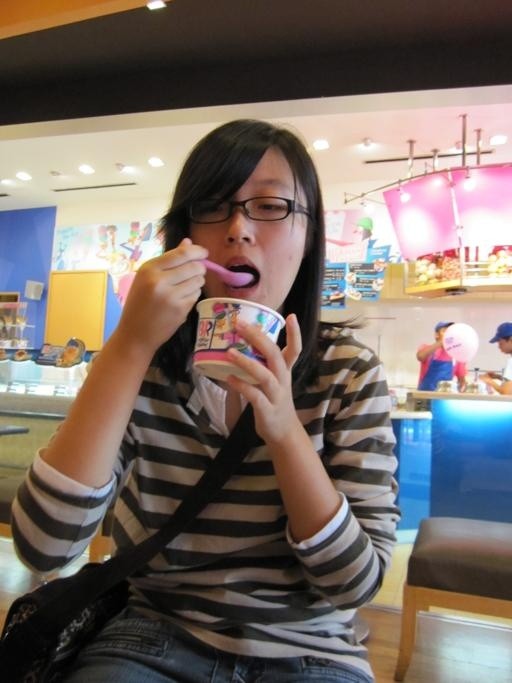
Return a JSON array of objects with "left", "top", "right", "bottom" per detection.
[
  {"left": 476, "top": 321, "right": 512, "bottom": 395},
  {"left": 414, "top": 320, "right": 467, "bottom": 393},
  {"left": 9, "top": 113, "right": 404, "bottom": 682}
]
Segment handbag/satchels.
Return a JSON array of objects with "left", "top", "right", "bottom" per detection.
[{"left": 1, "top": 556, "right": 128, "bottom": 681}]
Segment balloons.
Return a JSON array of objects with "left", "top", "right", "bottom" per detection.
[{"left": 443, "top": 321, "right": 479, "bottom": 363}]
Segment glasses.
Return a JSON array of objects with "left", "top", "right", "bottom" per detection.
[{"left": 186, "top": 195, "right": 314, "bottom": 225}]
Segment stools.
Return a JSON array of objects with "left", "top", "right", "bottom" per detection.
[{"left": 393, "top": 516, "right": 511, "bottom": 682}]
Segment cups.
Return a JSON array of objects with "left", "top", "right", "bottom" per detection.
[{"left": 193, "top": 297, "right": 286, "bottom": 386}]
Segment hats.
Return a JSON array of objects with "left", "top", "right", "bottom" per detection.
[
  {"left": 435, "top": 320, "right": 454, "bottom": 332},
  {"left": 489, "top": 320, "right": 511, "bottom": 343}
]
